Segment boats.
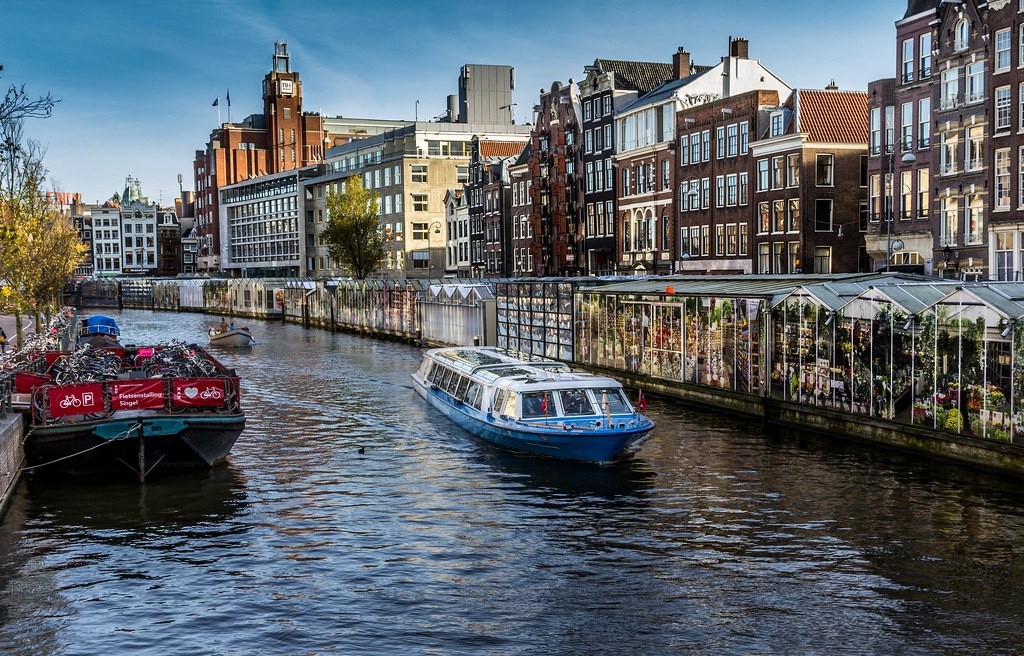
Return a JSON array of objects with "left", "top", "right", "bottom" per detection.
[
  {"left": 78, "top": 315, "right": 121, "bottom": 347},
  {"left": 208, "top": 328, "right": 255, "bottom": 346},
  {"left": 409, "top": 345, "right": 657, "bottom": 463}
]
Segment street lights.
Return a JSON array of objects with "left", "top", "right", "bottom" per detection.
[
  {"left": 588, "top": 248, "right": 595, "bottom": 277},
  {"left": 428, "top": 220, "right": 442, "bottom": 278},
  {"left": 678, "top": 174, "right": 699, "bottom": 274},
  {"left": 886, "top": 134, "right": 918, "bottom": 273}
]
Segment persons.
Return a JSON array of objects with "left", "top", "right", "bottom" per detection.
[
  {"left": 0, "top": 327, "right": 6, "bottom": 353},
  {"left": 209, "top": 318, "right": 234, "bottom": 336}
]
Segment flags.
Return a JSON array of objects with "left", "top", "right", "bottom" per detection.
[
  {"left": 638, "top": 393, "right": 646, "bottom": 411},
  {"left": 541, "top": 396, "right": 548, "bottom": 413}
]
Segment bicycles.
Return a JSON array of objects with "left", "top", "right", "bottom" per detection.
[{"left": 0, "top": 305, "right": 247, "bottom": 483}]
